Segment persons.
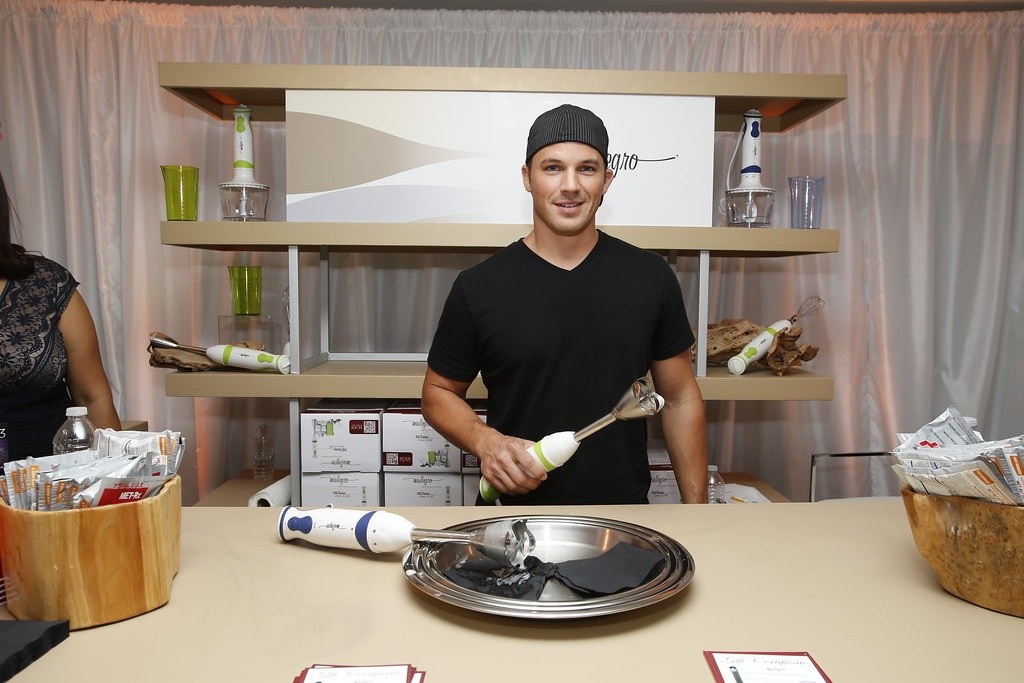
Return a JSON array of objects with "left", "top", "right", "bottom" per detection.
[
  {"left": 0, "top": 169, "right": 122, "bottom": 474},
  {"left": 421, "top": 104, "right": 714, "bottom": 505}
]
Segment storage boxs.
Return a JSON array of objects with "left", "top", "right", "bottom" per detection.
[
  {"left": 646, "top": 438, "right": 682, "bottom": 503},
  {"left": 217, "top": 315, "right": 282, "bottom": 356},
  {"left": 300, "top": 398, "right": 394, "bottom": 473},
  {"left": 461, "top": 475, "right": 480, "bottom": 507},
  {"left": 383, "top": 473, "right": 463, "bottom": 507},
  {"left": 301, "top": 473, "right": 385, "bottom": 508},
  {"left": 382, "top": 400, "right": 474, "bottom": 473},
  {"left": 461, "top": 402, "right": 487, "bottom": 475}
]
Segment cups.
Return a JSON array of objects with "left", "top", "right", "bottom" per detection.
[
  {"left": 227, "top": 265, "right": 261, "bottom": 314},
  {"left": 160, "top": 165, "right": 198, "bottom": 221},
  {"left": 427, "top": 451, "right": 436, "bottom": 464},
  {"left": 326, "top": 423, "right": 333, "bottom": 435},
  {"left": 788, "top": 176, "right": 823, "bottom": 230}
]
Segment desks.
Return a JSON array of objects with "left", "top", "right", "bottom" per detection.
[{"left": 0, "top": 494, "right": 1024, "bottom": 683}]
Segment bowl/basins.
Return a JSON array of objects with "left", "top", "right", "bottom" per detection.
[{"left": 900, "top": 484, "right": 1024, "bottom": 619}]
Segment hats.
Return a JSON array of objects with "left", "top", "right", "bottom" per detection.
[{"left": 525, "top": 103, "right": 609, "bottom": 169}]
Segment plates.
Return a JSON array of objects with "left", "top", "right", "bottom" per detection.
[{"left": 401, "top": 514, "right": 695, "bottom": 620}]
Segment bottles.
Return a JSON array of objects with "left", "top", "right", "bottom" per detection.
[
  {"left": 707, "top": 465, "right": 725, "bottom": 505},
  {"left": 53, "top": 406, "right": 97, "bottom": 454},
  {"left": 253, "top": 425, "right": 274, "bottom": 480}
]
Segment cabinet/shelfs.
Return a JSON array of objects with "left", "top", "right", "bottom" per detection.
[{"left": 157, "top": 62, "right": 849, "bottom": 508}]
